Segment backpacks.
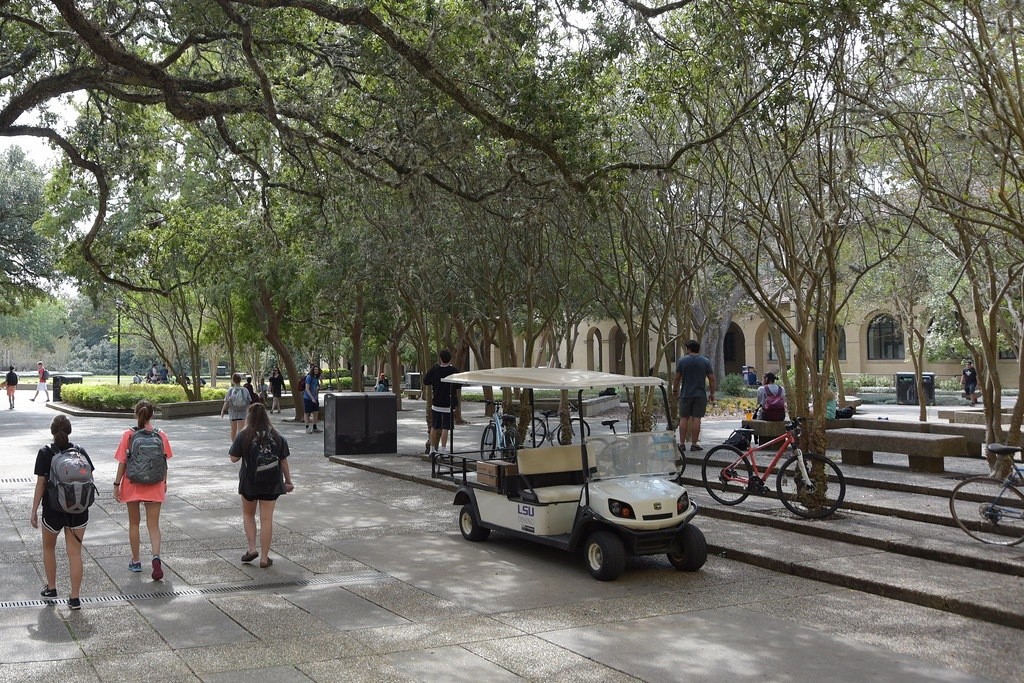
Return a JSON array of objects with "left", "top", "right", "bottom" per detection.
[
  {"left": 46, "top": 442, "right": 99, "bottom": 514},
  {"left": 240, "top": 426, "right": 282, "bottom": 494},
  {"left": 228, "top": 386, "right": 248, "bottom": 411},
  {"left": 39, "top": 368, "right": 49, "bottom": 380},
  {"left": 125, "top": 427, "right": 168, "bottom": 484},
  {"left": 161, "top": 368, "right": 168, "bottom": 380},
  {"left": 763, "top": 385, "right": 785, "bottom": 422},
  {"left": 297, "top": 373, "right": 314, "bottom": 391}
]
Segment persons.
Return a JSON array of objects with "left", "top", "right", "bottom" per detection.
[
  {"left": 29, "top": 361, "right": 49, "bottom": 402},
  {"left": 268, "top": 367, "right": 287, "bottom": 414},
  {"left": 302, "top": 365, "right": 323, "bottom": 434},
  {"left": 132, "top": 363, "right": 206, "bottom": 387},
  {"left": 752, "top": 372, "right": 786, "bottom": 444},
  {"left": 243, "top": 377, "right": 260, "bottom": 403},
  {"left": 960, "top": 361, "right": 977, "bottom": 407},
  {"left": 423, "top": 349, "right": 462, "bottom": 458},
  {"left": 30, "top": 415, "right": 94, "bottom": 610},
  {"left": 257, "top": 378, "right": 269, "bottom": 401},
  {"left": 221, "top": 374, "right": 253, "bottom": 442},
  {"left": 5, "top": 365, "right": 18, "bottom": 409},
  {"left": 379, "top": 373, "right": 389, "bottom": 391},
  {"left": 113, "top": 400, "right": 173, "bottom": 580},
  {"left": 808, "top": 371, "right": 837, "bottom": 420},
  {"left": 673, "top": 339, "right": 716, "bottom": 452},
  {"left": 229, "top": 402, "right": 294, "bottom": 568}
]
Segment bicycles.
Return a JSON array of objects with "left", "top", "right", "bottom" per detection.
[
  {"left": 477, "top": 399, "right": 591, "bottom": 461},
  {"left": 950, "top": 443, "right": 1024, "bottom": 545},
  {"left": 589, "top": 420, "right": 686, "bottom": 486},
  {"left": 702, "top": 417, "right": 846, "bottom": 520}
]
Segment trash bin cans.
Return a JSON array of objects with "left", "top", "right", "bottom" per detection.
[
  {"left": 321, "top": 392, "right": 399, "bottom": 458},
  {"left": 895, "top": 372, "right": 938, "bottom": 405},
  {"left": 51, "top": 375, "right": 83, "bottom": 402}
]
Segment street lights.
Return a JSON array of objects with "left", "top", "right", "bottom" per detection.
[{"left": 114, "top": 296, "right": 124, "bottom": 384}]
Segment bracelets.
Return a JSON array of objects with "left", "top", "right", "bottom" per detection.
[
  {"left": 114, "top": 482, "right": 120, "bottom": 486},
  {"left": 673, "top": 390, "right": 677, "bottom": 392}
]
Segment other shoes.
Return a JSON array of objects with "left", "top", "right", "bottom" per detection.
[
  {"left": 305, "top": 428, "right": 323, "bottom": 434},
  {"left": 427, "top": 447, "right": 454, "bottom": 460},
  {"left": 10, "top": 404, "right": 14, "bottom": 409},
  {"left": 970, "top": 399, "right": 977, "bottom": 406},
  {"left": 270, "top": 410, "right": 282, "bottom": 414},
  {"left": 29, "top": 399, "right": 50, "bottom": 402}
]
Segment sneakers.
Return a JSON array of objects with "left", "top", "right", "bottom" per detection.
[
  {"left": 128, "top": 559, "right": 141, "bottom": 572},
  {"left": 39, "top": 585, "right": 57, "bottom": 600},
  {"left": 677, "top": 443, "right": 704, "bottom": 451},
  {"left": 66, "top": 597, "right": 81, "bottom": 610},
  {"left": 151, "top": 553, "right": 164, "bottom": 580}
]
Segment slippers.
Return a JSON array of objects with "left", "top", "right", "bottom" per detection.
[
  {"left": 260, "top": 557, "right": 272, "bottom": 568},
  {"left": 241, "top": 549, "right": 258, "bottom": 561}
]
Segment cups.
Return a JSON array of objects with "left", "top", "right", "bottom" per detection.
[{"left": 745, "top": 409, "right": 752, "bottom": 420}]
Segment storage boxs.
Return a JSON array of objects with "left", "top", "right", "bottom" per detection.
[{"left": 475, "top": 458, "right": 517, "bottom": 490}]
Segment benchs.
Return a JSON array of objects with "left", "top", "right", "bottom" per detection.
[
  {"left": 514, "top": 442, "right": 602, "bottom": 505},
  {"left": 746, "top": 403, "right": 1024, "bottom": 472}
]
